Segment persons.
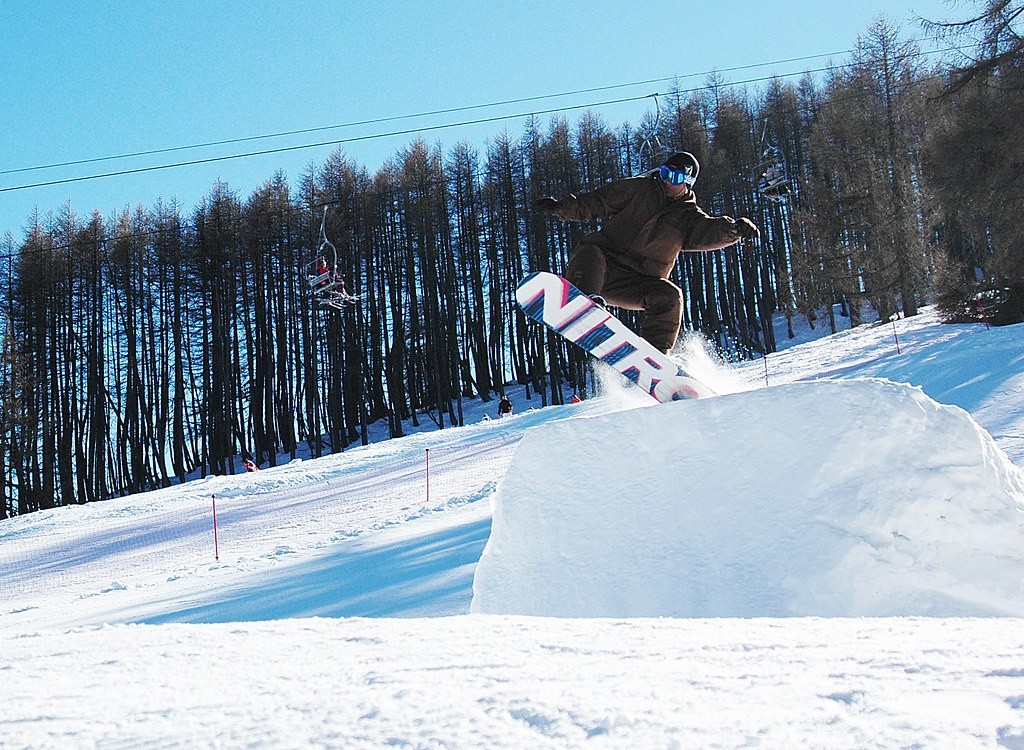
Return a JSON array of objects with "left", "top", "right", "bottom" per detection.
[
  {"left": 497, "top": 395, "right": 513, "bottom": 418},
  {"left": 571, "top": 393, "right": 580, "bottom": 403},
  {"left": 532, "top": 152, "right": 760, "bottom": 356},
  {"left": 243, "top": 458, "right": 259, "bottom": 472}
]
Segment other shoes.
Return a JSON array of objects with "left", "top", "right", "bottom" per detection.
[{"left": 588, "top": 294, "right": 608, "bottom": 308}]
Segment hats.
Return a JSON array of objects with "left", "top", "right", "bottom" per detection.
[{"left": 665, "top": 151, "right": 700, "bottom": 187}]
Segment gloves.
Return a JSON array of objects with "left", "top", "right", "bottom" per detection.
[
  {"left": 730, "top": 216, "right": 761, "bottom": 240},
  {"left": 530, "top": 197, "right": 564, "bottom": 216}
]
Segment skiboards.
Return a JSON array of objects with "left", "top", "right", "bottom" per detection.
[{"left": 315, "top": 294, "right": 367, "bottom": 310}]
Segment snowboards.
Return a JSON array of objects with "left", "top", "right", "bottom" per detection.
[{"left": 514, "top": 269, "right": 717, "bottom": 406}]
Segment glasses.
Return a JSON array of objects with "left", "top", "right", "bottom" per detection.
[{"left": 659, "top": 165, "right": 687, "bottom": 185}]
